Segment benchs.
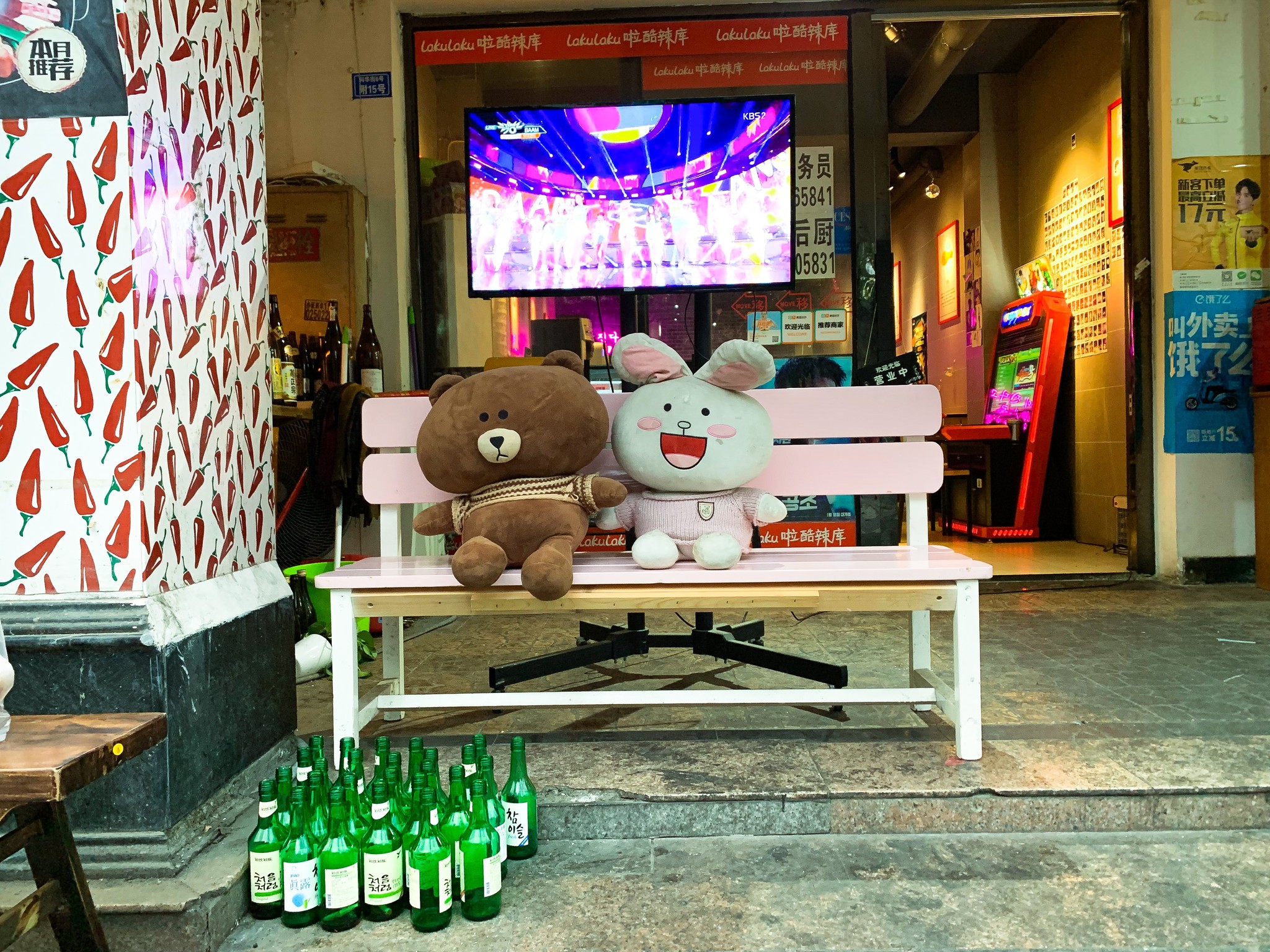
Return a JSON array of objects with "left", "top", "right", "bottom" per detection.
[
  {"left": 314, "top": 385, "right": 995, "bottom": 760},
  {"left": 0, "top": 711, "right": 168, "bottom": 952}
]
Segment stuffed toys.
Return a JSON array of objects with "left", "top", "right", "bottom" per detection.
[
  {"left": 411, "top": 349, "right": 629, "bottom": 601},
  {"left": 595, "top": 332, "right": 788, "bottom": 570}
]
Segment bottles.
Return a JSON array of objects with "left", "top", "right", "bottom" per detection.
[
  {"left": 268, "top": 288, "right": 386, "bottom": 409},
  {"left": 242, "top": 733, "right": 541, "bottom": 931},
  {"left": 284, "top": 569, "right": 323, "bottom": 656}
]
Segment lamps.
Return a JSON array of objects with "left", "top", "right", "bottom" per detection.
[
  {"left": 892, "top": 160, "right": 906, "bottom": 177},
  {"left": 885, "top": 24, "right": 902, "bottom": 43},
  {"left": 924, "top": 184, "right": 939, "bottom": 199}
]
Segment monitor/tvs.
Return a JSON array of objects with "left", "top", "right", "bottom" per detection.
[{"left": 466, "top": 93, "right": 793, "bottom": 296}]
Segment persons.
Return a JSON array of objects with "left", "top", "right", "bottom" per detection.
[
  {"left": 1202, "top": 366, "right": 1224, "bottom": 401},
  {"left": 961, "top": 226, "right": 1049, "bottom": 347},
  {"left": 772, "top": 356, "right": 847, "bottom": 445},
  {"left": 471, "top": 170, "right": 790, "bottom": 290},
  {"left": 1209, "top": 178, "right": 1269, "bottom": 268}
]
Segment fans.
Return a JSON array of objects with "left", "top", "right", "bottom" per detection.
[{"left": 273, "top": 415, "right": 357, "bottom": 569}]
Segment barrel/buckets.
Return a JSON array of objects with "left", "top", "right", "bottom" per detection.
[
  {"left": 285, "top": 554, "right": 384, "bottom": 646},
  {"left": 294, "top": 633, "right": 332, "bottom": 677}
]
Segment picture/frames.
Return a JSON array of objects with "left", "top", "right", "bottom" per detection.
[
  {"left": 895, "top": 260, "right": 902, "bottom": 342},
  {"left": 1108, "top": 96, "right": 1124, "bottom": 227},
  {"left": 935, "top": 219, "right": 960, "bottom": 325}
]
